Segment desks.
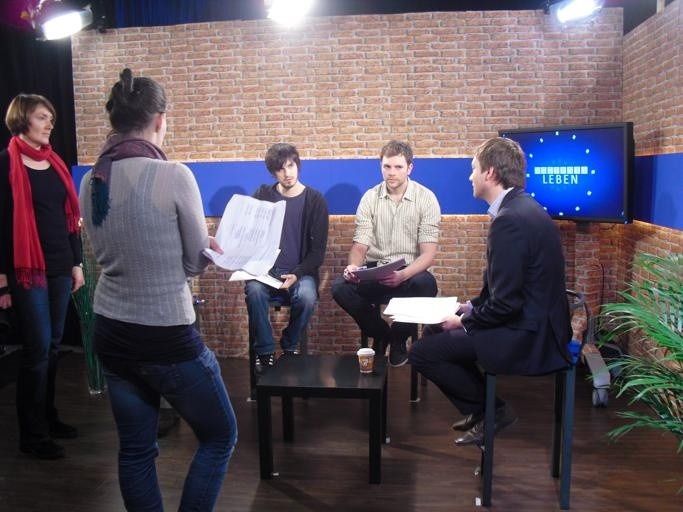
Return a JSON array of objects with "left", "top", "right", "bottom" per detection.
[{"left": 254, "top": 354, "right": 389, "bottom": 485}]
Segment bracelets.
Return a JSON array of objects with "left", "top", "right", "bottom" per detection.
[{"left": 0, "top": 285, "right": 12, "bottom": 296}]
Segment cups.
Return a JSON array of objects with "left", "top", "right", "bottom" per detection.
[{"left": 355, "top": 347, "right": 375, "bottom": 374}]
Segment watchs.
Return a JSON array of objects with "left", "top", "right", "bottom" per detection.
[{"left": 73, "top": 262, "right": 84, "bottom": 267}]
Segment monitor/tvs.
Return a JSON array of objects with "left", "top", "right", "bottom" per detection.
[{"left": 499, "top": 122, "right": 633, "bottom": 225}]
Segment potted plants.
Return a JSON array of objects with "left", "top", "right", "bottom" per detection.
[{"left": 68, "top": 219, "right": 110, "bottom": 394}]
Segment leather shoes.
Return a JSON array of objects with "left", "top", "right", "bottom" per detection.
[{"left": 452, "top": 402, "right": 519, "bottom": 446}]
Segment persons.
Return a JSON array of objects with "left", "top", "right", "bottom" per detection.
[
  {"left": 330, "top": 137, "right": 442, "bottom": 367},
  {"left": 244, "top": 142, "right": 329, "bottom": 375},
  {"left": 0, "top": 89, "right": 86, "bottom": 460},
  {"left": 407, "top": 137, "right": 572, "bottom": 447},
  {"left": 79, "top": 64, "right": 239, "bottom": 512}
]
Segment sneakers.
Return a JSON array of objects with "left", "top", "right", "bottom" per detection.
[
  {"left": 37, "top": 414, "right": 78, "bottom": 438},
  {"left": 19, "top": 434, "right": 66, "bottom": 461},
  {"left": 254, "top": 340, "right": 276, "bottom": 378},
  {"left": 371, "top": 320, "right": 391, "bottom": 363},
  {"left": 388, "top": 323, "right": 409, "bottom": 368},
  {"left": 279, "top": 346, "right": 299, "bottom": 359}
]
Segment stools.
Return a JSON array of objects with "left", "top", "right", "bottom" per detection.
[
  {"left": 153, "top": 296, "right": 208, "bottom": 426},
  {"left": 361, "top": 300, "right": 428, "bottom": 403},
  {"left": 247, "top": 299, "right": 307, "bottom": 403},
  {"left": 482, "top": 366, "right": 576, "bottom": 511}
]
